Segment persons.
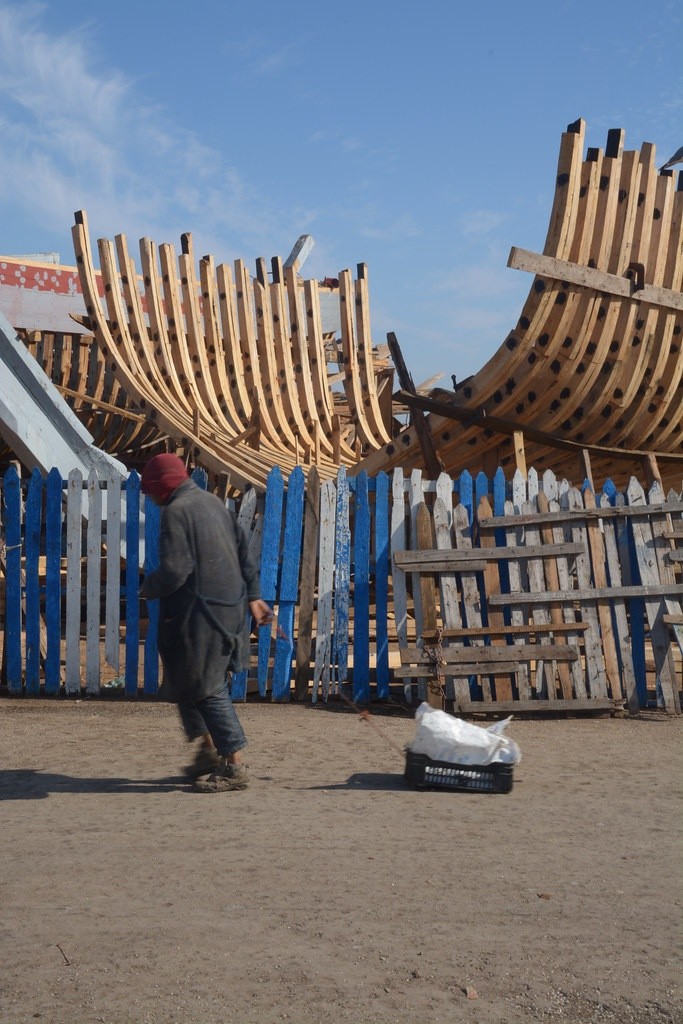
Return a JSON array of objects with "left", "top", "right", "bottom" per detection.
[{"left": 136, "top": 453, "right": 279, "bottom": 794}]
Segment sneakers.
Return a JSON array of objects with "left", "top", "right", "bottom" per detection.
[
  {"left": 183, "top": 743, "right": 220, "bottom": 777},
  {"left": 193, "top": 762, "right": 249, "bottom": 793}
]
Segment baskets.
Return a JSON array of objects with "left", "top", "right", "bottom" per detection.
[{"left": 401, "top": 750, "right": 514, "bottom": 795}]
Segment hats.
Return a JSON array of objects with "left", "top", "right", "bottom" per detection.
[{"left": 140, "top": 453, "right": 187, "bottom": 494}]
